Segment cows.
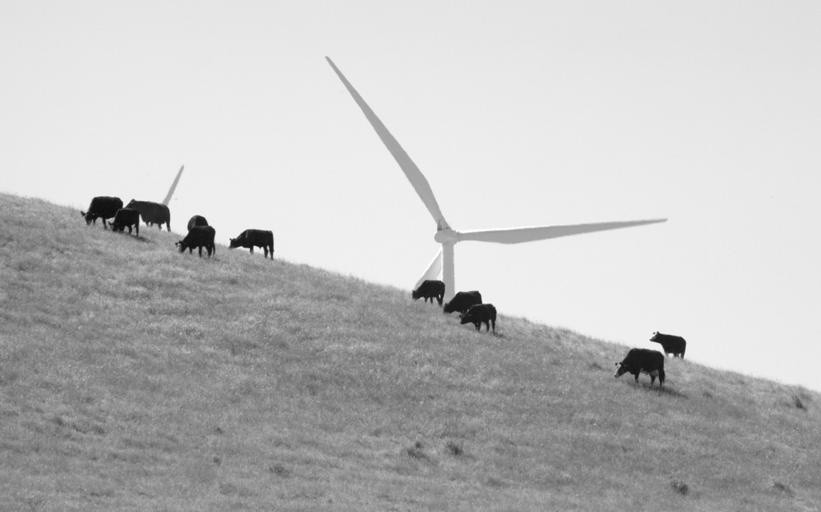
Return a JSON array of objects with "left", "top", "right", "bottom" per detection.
[
  {"left": 412, "top": 280, "right": 445, "bottom": 306},
  {"left": 175, "top": 226, "right": 216, "bottom": 259},
  {"left": 187, "top": 215, "right": 208, "bottom": 231},
  {"left": 650, "top": 330, "right": 686, "bottom": 360},
  {"left": 458, "top": 304, "right": 497, "bottom": 334},
  {"left": 124, "top": 199, "right": 171, "bottom": 233},
  {"left": 108, "top": 208, "right": 139, "bottom": 237},
  {"left": 443, "top": 291, "right": 483, "bottom": 315},
  {"left": 228, "top": 229, "right": 274, "bottom": 260},
  {"left": 613, "top": 348, "right": 666, "bottom": 389},
  {"left": 80, "top": 196, "right": 124, "bottom": 230}
]
